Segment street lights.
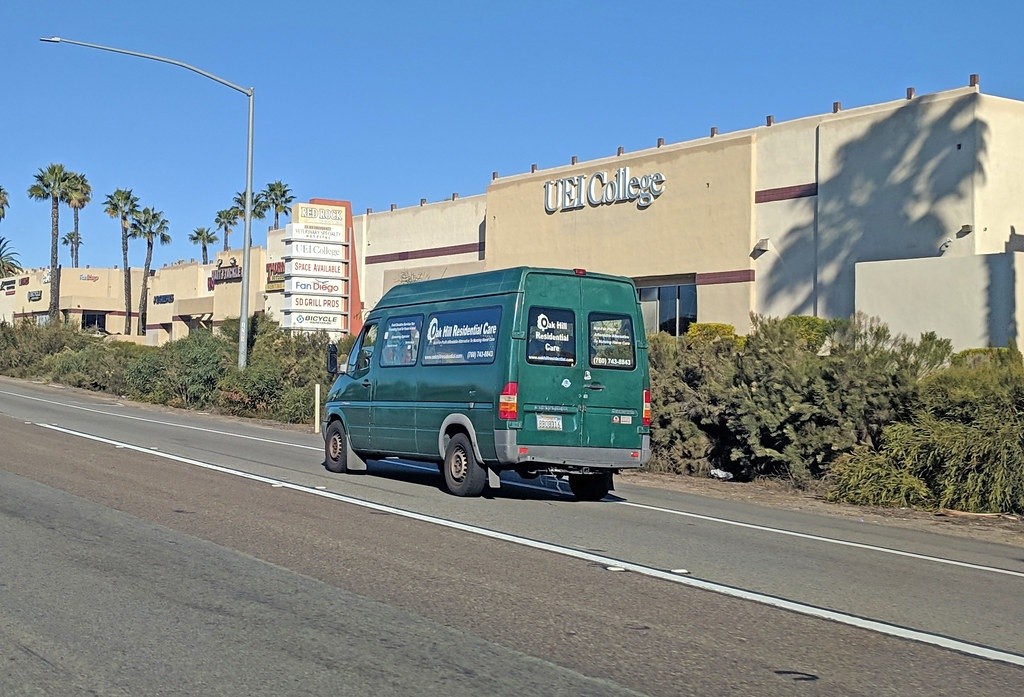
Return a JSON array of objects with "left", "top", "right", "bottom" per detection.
[{"left": 40, "top": 35, "right": 254, "bottom": 370}]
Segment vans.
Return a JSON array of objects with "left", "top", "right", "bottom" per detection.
[{"left": 323, "top": 265, "right": 653, "bottom": 500}]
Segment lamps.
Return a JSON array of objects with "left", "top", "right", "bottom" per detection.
[
  {"left": 755, "top": 239, "right": 768, "bottom": 250},
  {"left": 264, "top": 296, "right": 267, "bottom": 299}
]
